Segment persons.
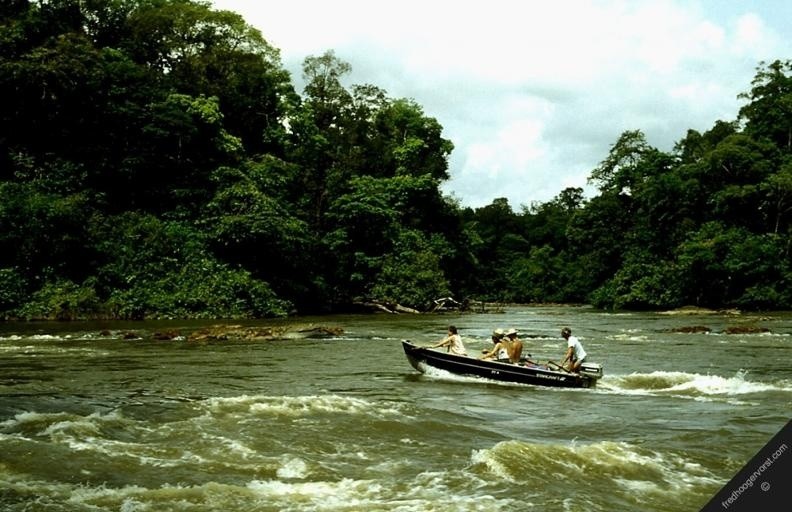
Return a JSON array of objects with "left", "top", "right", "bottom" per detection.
[
  {"left": 426, "top": 325, "right": 467, "bottom": 356},
  {"left": 505, "top": 327, "right": 524, "bottom": 364},
  {"left": 481, "top": 328, "right": 514, "bottom": 365},
  {"left": 476, "top": 334, "right": 510, "bottom": 364},
  {"left": 558, "top": 327, "right": 587, "bottom": 372}
]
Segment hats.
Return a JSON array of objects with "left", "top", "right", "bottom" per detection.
[
  {"left": 505, "top": 328, "right": 519, "bottom": 337},
  {"left": 492, "top": 327, "right": 506, "bottom": 337}
]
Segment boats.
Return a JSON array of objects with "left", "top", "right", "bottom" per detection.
[{"left": 401, "top": 334, "right": 603, "bottom": 390}]
